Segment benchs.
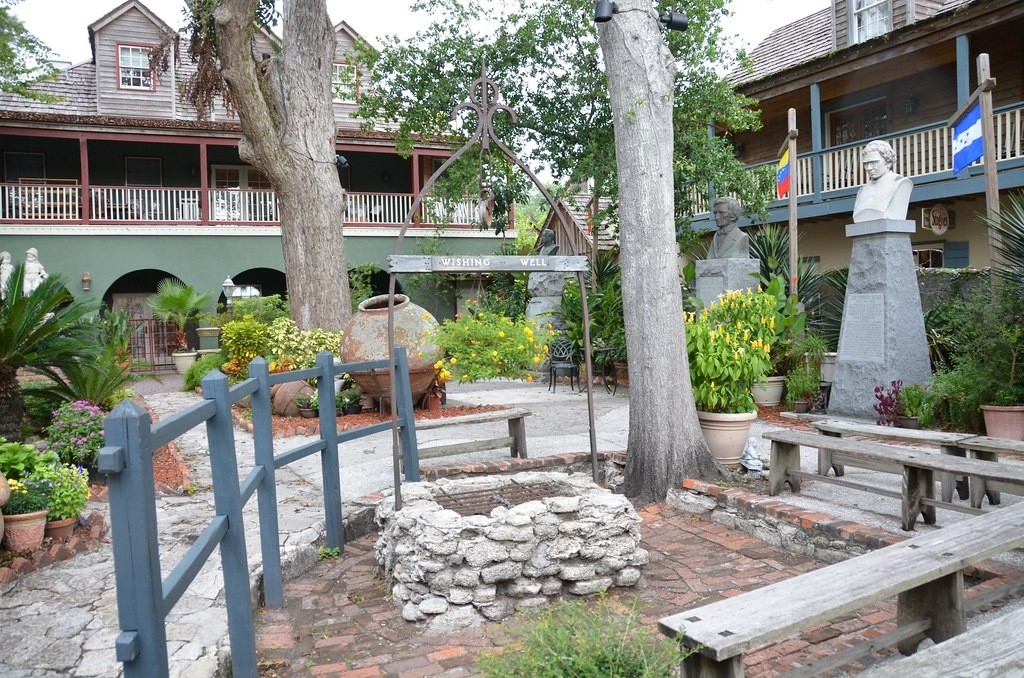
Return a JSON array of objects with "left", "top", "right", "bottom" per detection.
[
  {"left": 397, "top": 408, "right": 533, "bottom": 474},
  {"left": 658, "top": 419, "right": 1024, "bottom": 678}
]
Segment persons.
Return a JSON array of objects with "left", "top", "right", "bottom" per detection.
[
  {"left": 852, "top": 140, "right": 914, "bottom": 224},
  {"left": 705, "top": 197, "right": 750, "bottom": 259},
  {"left": 541, "top": 229, "right": 559, "bottom": 255}
]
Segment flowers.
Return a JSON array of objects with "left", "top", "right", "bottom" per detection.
[
  {"left": 875, "top": 380, "right": 934, "bottom": 428},
  {"left": 0, "top": 397, "right": 110, "bottom": 523},
  {"left": 799, "top": 390, "right": 817, "bottom": 411},
  {"left": 683, "top": 257, "right": 806, "bottom": 414},
  {"left": 291, "top": 389, "right": 365, "bottom": 410},
  {"left": 416, "top": 301, "right": 562, "bottom": 386}
]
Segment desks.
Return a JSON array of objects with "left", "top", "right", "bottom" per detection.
[{"left": 580, "top": 348, "right": 616, "bottom": 395}]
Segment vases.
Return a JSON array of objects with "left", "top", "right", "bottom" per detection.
[
  {"left": 342, "top": 404, "right": 364, "bottom": 415},
  {"left": 898, "top": 416, "right": 920, "bottom": 429},
  {"left": 300, "top": 408, "right": 319, "bottom": 418},
  {"left": 804, "top": 352, "right": 837, "bottom": 382},
  {"left": 794, "top": 401, "right": 809, "bottom": 413},
  {"left": 335, "top": 408, "right": 341, "bottom": 417},
  {"left": 750, "top": 376, "right": 784, "bottom": 407},
  {"left": 271, "top": 380, "right": 316, "bottom": 418},
  {"left": 2, "top": 510, "right": 77, "bottom": 554},
  {"left": 697, "top": 411, "right": 757, "bottom": 466},
  {"left": 339, "top": 294, "right": 445, "bottom": 410}
]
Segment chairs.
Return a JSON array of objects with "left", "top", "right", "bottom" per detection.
[{"left": 547, "top": 339, "right": 631, "bottom": 397}]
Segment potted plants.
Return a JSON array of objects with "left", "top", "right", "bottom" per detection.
[
  {"left": 955, "top": 277, "right": 1024, "bottom": 442},
  {"left": 145, "top": 278, "right": 213, "bottom": 375}
]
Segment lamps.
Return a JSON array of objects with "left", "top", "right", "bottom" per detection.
[
  {"left": 734, "top": 142, "right": 744, "bottom": 157},
  {"left": 81, "top": 272, "right": 92, "bottom": 291},
  {"left": 906, "top": 94, "right": 920, "bottom": 116}
]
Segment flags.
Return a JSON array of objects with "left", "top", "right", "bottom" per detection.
[
  {"left": 778, "top": 149, "right": 790, "bottom": 199},
  {"left": 953, "top": 103, "right": 983, "bottom": 176}
]
